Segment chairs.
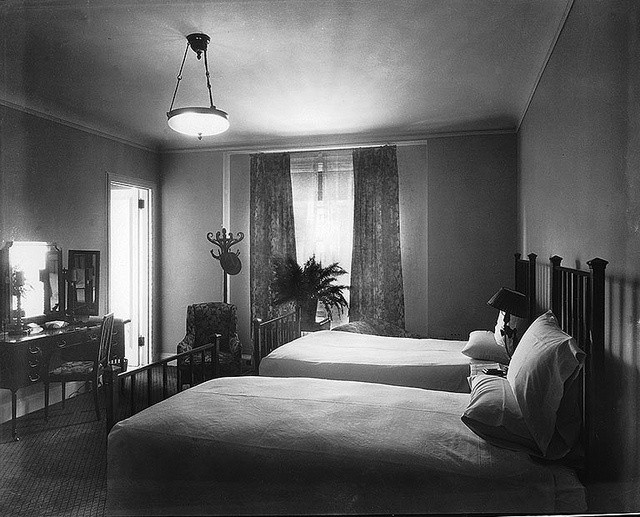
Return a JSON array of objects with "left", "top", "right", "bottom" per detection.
[{"left": 43, "top": 311, "right": 115, "bottom": 423}]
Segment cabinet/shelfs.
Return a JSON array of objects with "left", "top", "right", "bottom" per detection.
[{"left": 1, "top": 316, "right": 129, "bottom": 441}]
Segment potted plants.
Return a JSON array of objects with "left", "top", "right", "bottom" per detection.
[{"left": 269, "top": 251, "right": 353, "bottom": 331}]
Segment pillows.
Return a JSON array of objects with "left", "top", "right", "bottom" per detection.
[
  {"left": 493, "top": 309, "right": 523, "bottom": 347},
  {"left": 506, "top": 308, "right": 586, "bottom": 458},
  {"left": 462, "top": 325, "right": 512, "bottom": 365},
  {"left": 460, "top": 372, "right": 574, "bottom": 462}
]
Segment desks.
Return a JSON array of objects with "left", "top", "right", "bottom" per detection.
[{"left": 301, "top": 316, "right": 331, "bottom": 332}]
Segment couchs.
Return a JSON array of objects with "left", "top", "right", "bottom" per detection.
[{"left": 178, "top": 300, "right": 242, "bottom": 388}]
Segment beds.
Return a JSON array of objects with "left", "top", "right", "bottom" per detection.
[
  {"left": 249, "top": 249, "right": 537, "bottom": 392},
  {"left": 106, "top": 254, "right": 609, "bottom": 516}
]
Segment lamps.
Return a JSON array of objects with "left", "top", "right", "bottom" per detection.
[
  {"left": 165, "top": 32, "right": 231, "bottom": 141},
  {"left": 482, "top": 286, "right": 529, "bottom": 376}
]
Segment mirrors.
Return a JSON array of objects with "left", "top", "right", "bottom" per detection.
[
  {"left": 3, "top": 239, "right": 63, "bottom": 324},
  {"left": 68, "top": 249, "right": 100, "bottom": 316}
]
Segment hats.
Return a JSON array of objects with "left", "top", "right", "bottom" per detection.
[{"left": 220, "top": 252, "right": 242, "bottom": 275}]
